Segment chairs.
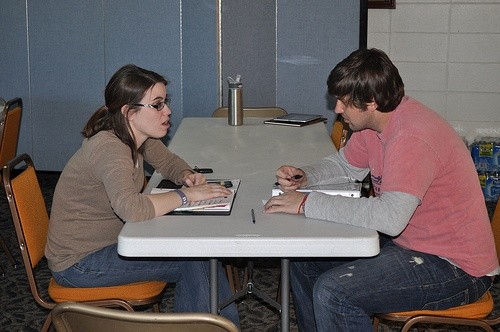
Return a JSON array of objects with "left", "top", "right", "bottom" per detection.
[
  {"left": 3, "top": 154, "right": 167, "bottom": 332},
  {"left": 212, "top": 107, "right": 289, "bottom": 302},
  {"left": 372, "top": 197, "right": 500, "bottom": 332},
  {"left": 0, "top": 97, "right": 24, "bottom": 268},
  {"left": 117, "top": 118, "right": 380, "bottom": 332},
  {"left": 50, "top": 302, "right": 239, "bottom": 332},
  {"left": 329, "top": 114, "right": 348, "bottom": 150}
]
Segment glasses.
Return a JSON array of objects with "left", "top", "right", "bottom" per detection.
[{"left": 128, "top": 98, "right": 171, "bottom": 111}]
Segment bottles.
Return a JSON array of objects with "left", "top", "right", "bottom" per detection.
[{"left": 459, "top": 126, "right": 500, "bottom": 204}]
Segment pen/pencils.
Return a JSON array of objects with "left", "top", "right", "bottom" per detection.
[
  {"left": 250, "top": 208, "right": 256, "bottom": 225},
  {"left": 272, "top": 174, "right": 303, "bottom": 187}
]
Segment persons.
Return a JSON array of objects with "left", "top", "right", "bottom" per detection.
[
  {"left": 43, "top": 65, "right": 242, "bottom": 329},
  {"left": 266, "top": 46, "right": 498, "bottom": 332}
]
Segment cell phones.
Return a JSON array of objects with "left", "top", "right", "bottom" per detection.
[{"left": 193, "top": 168, "right": 212, "bottom": 173}]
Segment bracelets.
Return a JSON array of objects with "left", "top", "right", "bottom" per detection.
[
  {"left": 170, "top": 190, "right": 188, "bottom": 206},
  {"left": 298, "top": 192, "right": 310, "bottom": 215}
]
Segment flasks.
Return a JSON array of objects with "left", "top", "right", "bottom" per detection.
[{"left": 228, "top": 82, "right": 244, "bottom": 126}]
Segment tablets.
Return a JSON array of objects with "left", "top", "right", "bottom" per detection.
[{"left": 273, "top": 114, "right": 321, "bottom": 124}]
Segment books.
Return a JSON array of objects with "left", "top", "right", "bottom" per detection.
[
  {"left": 264, "top": 113, "right": 327, "bottom": 127},
  {"left": 270, "top": 179, "right": 362, "bottom": 199},
  {"left": 152, "top": 176, "right": 242, "bottom": 215}
]
